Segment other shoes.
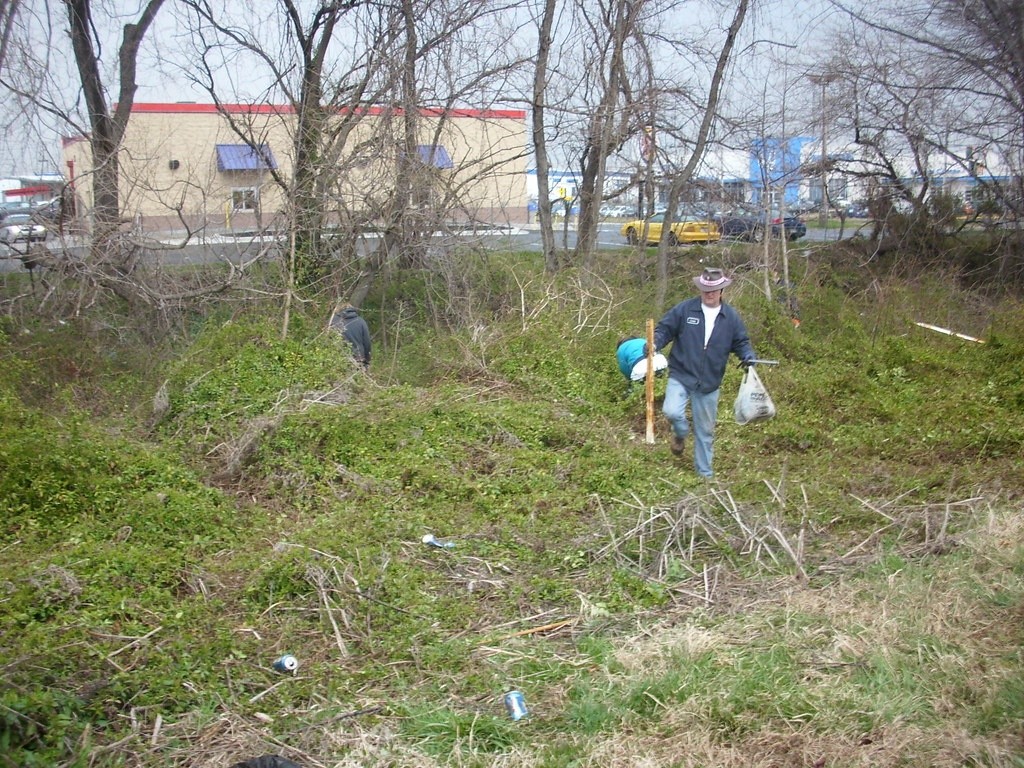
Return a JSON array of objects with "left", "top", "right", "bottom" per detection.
[{"left": 671, "top": 436, "right": 685, "bottom": 455}]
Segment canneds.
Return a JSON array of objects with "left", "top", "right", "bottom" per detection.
[
  {"left": 273, "top": 654, "right": 298, "bottom": 673},
  {"left": 506, "top": 690, "right": 527, "bottom": 719},
  {"left": 422, "top": 534, "right": 456, "bottom": 548}
]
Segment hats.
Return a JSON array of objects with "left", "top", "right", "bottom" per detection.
[{"left": 693, "top": 267, "right": 732, "bottom": 293}]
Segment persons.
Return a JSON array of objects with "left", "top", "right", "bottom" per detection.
[
  {"left": 773, "top": 270, "right": 800, "bottom": 326},
  {"left": 329, "top": 302, "right": 372, "bottom": 374},
  {"left": 617, "top": 335, "right": 668, "bottom": 401},
  {"left": 642, "top": 267, "right": 756, "bottom": 478}
]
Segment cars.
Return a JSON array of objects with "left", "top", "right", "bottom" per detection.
[
  {"left": 620, "top": 211, "right": 719, "bottom": 247},
  {"left": 0, "top": 214, "right": 48, "bottom": 243},
  {"left": 718, "top": 208, "right": 807, "bottom": 243}
]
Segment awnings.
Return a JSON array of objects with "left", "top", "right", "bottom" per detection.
[
  {"left": 217, "top": 144, "right": 278, "bottom": 171},
  {"left": 399, "top": 145, "right": 454, "bottom": 170}
]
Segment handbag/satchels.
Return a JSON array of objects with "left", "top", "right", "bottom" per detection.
[{"left": 734, "top": 366, "right": 776, "bottom": 425}]
